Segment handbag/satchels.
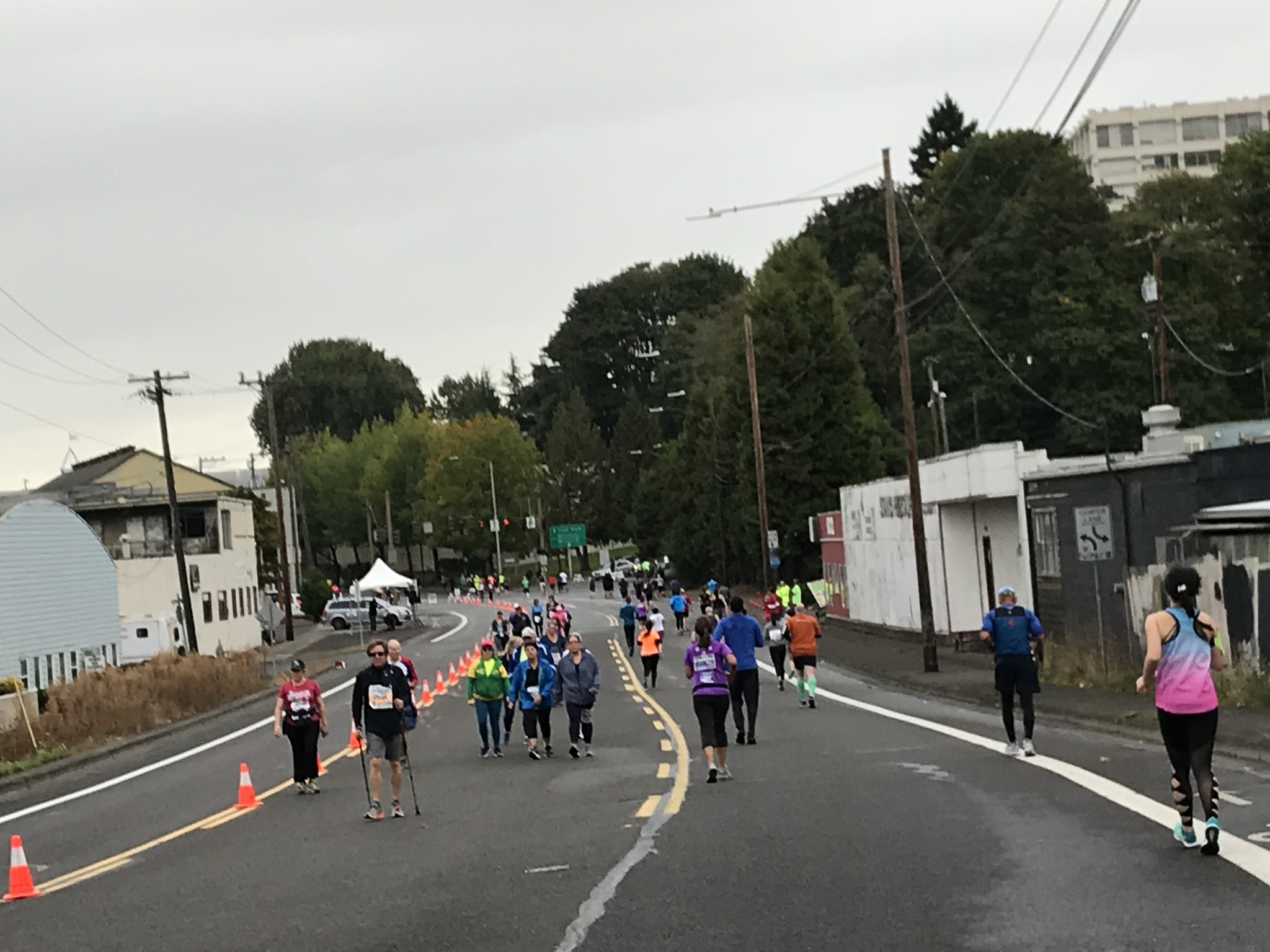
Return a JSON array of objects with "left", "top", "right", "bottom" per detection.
[{"left": 404, "top": 704, "right": 417, "bottom": 731}]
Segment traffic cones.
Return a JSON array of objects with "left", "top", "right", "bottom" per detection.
[
  {"left": 464, "top": 636, "right": 485, "bottom": 667},
  {"left": 233, "top": 762, "right": 264, "bottom": 809},
  {"left": 347, "top": 717, "right": 365, "bottom": 749},
  {"left": 411, "top": 689, "right": 417, "bottom": 707},
  {"left": 447, "top": 662, "right": 459, "bottom": 686},
  {"left": 462, "top": 595, "right": 523, "bottom": 612},
  {"left": 435, "top": 670, "right": 447, "bottom": 696},
  {"left": 317, "top": 751, "right": 328, "bottom": 777},
  {"left": 457, "top": 656, "right": 467, "bottom": 677},
  {"left": 419, "top": 679, "right": 434, "bottom": 707},
  {"left": 1, "top": 834, "right": 40, "bottom": 901}
]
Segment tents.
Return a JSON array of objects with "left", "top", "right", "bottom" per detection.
[{"left": 350, "top": 557, "right": 417, "bottom": 589}]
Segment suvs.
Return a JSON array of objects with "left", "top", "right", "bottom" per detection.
[{"left": 320, "top": 596, "right": 413, "bottom": 631}]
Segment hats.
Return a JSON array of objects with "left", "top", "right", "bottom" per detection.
[
  {"left": 522, "top": 627, "right": 537, "bottom": 638},
  {"left": 997, "top": 585, "right": 1014, "bottom": 595},
  {"left": 290, "top": 659, "right": 305, "bottom": 672}
]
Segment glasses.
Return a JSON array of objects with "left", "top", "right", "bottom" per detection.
[{"left": 369, "top": 650, "right": 386, "bottom": 657}]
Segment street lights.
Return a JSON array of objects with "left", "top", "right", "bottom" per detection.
[{"left": 448, "top": 456, "right": 503, "bottom": 575}]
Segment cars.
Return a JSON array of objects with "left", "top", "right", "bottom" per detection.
[{"left": 590, "top": 559, "right": 636, "bottom": 580}]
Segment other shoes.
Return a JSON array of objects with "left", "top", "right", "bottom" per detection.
[
  {"left": 295, "top": 779, "right": 321, "bottom": 794},
  {"left": 364, "top": 804, "right": 384, "bottom": 822},
  {"left": 717, "top": 768, "right": 732, "bottom": 780},
  {"left": 493, "top": 747, "right": 504, "bottom": 758},
  {"left": 644, "top": 681, "right": 647, "bottom": 689},
  {"left": 504, "top": 733, "right": 510, "bottom": 745},
  {"left": 706, "top": 762, "right": 718, "bottom": 783},
  {"left": 400, "top": 761, "right": 409, "bottom": 769},
  {"left": 789, "top": 670, "right": 795, "bottom": 679},
  {"left": 629, "top": 648, "right": 633, "bottom": 657},
  {"left": 808, "top": 696, "right": 816, "bottom": 709},
  {"left": 543, "top": 740, "right": 554, "bottom": 758},
  {"left": 1005, "top": 740, "right": 1020, "bottom": 757},
  {"left": 778, "top": 680, "right": 785, "bottom": 692},
  {"left": 1173, "top": 822, "right": 1200, "bottom": 848},
  {"left": 558, "top": 701, "right": 563, "bottom": 707},
  {"left": 391, "top": 805, "right": 405, "bottom": 819},
  {"left": 799, "top": 699, "right": 807, "bottom": 708},
  {"left": 569, "top": 744, "right": 581, "bottom": 758},
  {"left": 747, "top": 731, "right": 757, "bottom": 745},
  {"left": 736, "top": 730, "right": 746, "bottom": 745},
  {"left": 1200, "top": 816, "right": 1220, "bottom": 855},
  {"left": 528, "top": 745, "right": 542, "bottom": 760},
  {"left": 652, "top": 682, "right": 655, "bottom": 688},
  {"left": 1023, "top": 738, "right": 1036, "bottom": 757},
  {"left": 523, "top": 738, "right": 530, "bottom": 745},
  {"left": 585, "top": 744, "right": 594, "bottom": 757},
  {"left": 479, "top": 746, "right": 490, "bottom": 759}
]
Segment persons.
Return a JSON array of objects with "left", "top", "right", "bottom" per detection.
[
  {"left": 685, "top": 617, "right": 737, "bottom": 783},
  {"left": 713, "top": 597, "right": 764, "bottom": 745},
  {"left": 670, "top": 575, "right": 729, "bottom": 635},
  {"left": 466, "top": 638, "right": 509, "bottom": 757},
  {"left": 353, "top": 582, "right": 425, "bottom": 631},
  {"left": 587, "top": 556, "right": 670, "bottom": 606},
  {"left": 1135, "top": 566, "right": 1227, "bottom": 856},
  {"left": 638, "top": 621, "right": 661, "bottom": 689},
  {"left": 783, "top": 603, "right": 821, "bottom": 709},
  {"left": 763, "top": 579, "right": 802, "bottom": 691},
  {"left": 274, "top": 659, "right": 328, "bottom": 793},
  {"left": 521, "top": 569, "right": 570, "bottom": 598},
  {"left": 980, "top": 586, "right": 1044, "bottom": 756},
  {"left": 494, "top": 596, "right": 573, "bottom": 741},
  {"left": 508, "top": 642, "right": 555, "bottom": 758},
  {"left": 353, "top": 638, "right": 419, "bottom": 822},
  {"left": 441, "top": 570, "right": 507, "bottom": 603},
  {"left": 619, "top": 597, "right": 663, "bottom": 656},
  {"left": 556, "top": 635, "right": 598, "bottom": 755}
]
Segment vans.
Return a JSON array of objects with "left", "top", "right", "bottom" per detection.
[{"left": 265, "top": 592, "right": 306, "bottom": 620}]
